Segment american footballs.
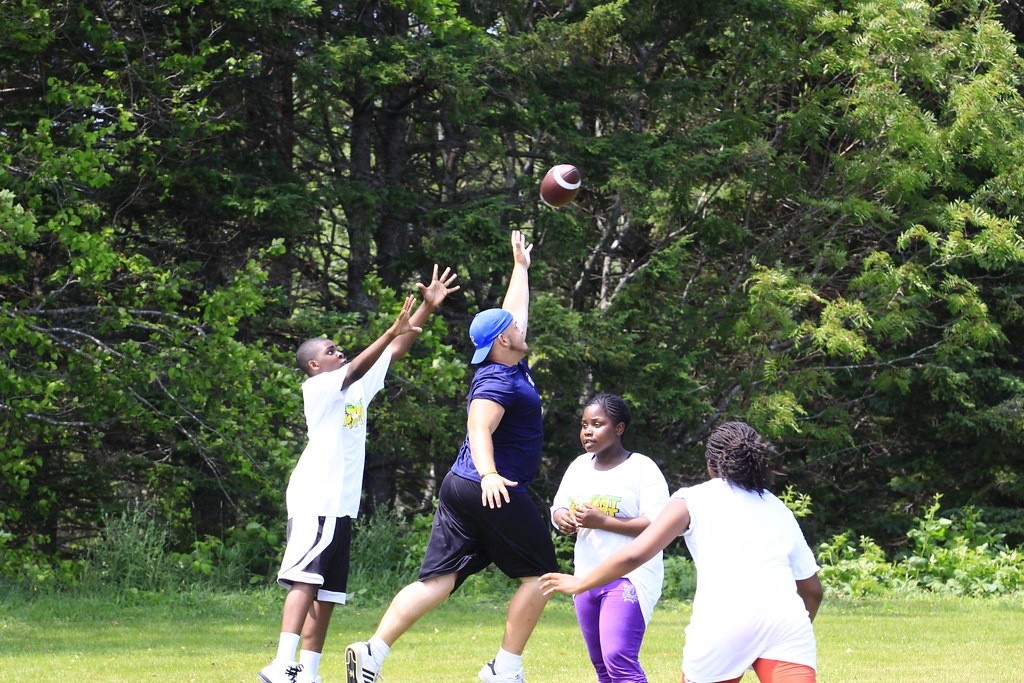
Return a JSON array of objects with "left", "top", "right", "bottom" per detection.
[{"left": 540, "top": 163, "right": 582, "bottom": 209}]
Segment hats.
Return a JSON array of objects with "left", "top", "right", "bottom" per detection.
[{"left": 469, "top": 308, "right": 513, "bottom": 364}]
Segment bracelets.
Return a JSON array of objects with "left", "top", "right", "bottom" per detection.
[{"left": 480, "top": 471, "right": 499, "bottom": 480}]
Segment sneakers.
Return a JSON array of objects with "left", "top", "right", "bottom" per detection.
[
  {"left": 257, "top": 661, "right": 323, "bottom": 683},
  {"left": 477, "top": 659, "right": 525, "bottom": 683},
  {"left": 345, "top": 642, "right": 380, "bottom": 683}
]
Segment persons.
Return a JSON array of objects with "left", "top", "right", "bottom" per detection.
[
  {"left": 539, "top": 422, "right": 824, "bottom": 682},
  {"left": 346, "top": 229, "right": 558, "bottom": 683},
  {"left": 260, "top": 262, "right": 459, "bottom": 683},
  {"left": 551, "top": 393, "right": 669, "bottom": 683}
]
